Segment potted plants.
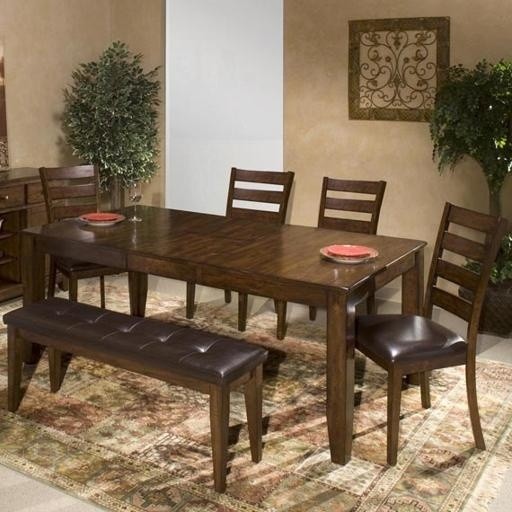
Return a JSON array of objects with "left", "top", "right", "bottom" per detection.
[
  {"left": 421, "top": 56, "right": 511, "bottom": 340},
  {"left": 60, "top": 37, "right": 162, "bottom": 279}
]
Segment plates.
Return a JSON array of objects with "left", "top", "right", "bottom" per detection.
[
  {"left": 79, "top": 212, "right": 126, "bottom": 226},
  {"left": 319, "top": 244, "right": 380, "bottom": 265}
]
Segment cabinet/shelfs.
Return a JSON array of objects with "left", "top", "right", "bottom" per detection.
[{"left": 0, "top": 165, "right": 73, "bottom": 304}]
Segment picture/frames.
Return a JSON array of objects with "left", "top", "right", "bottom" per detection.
[{"left": 344, "top": 13, "right": 453, "bottom": 124}]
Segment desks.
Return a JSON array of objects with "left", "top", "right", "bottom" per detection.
[{"left": 17, "top": 204, "right": 428, "bottom": 468}]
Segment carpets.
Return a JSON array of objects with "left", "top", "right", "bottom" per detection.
[{"left": 1, "top": 275, "right": 511, "bottom": 511}]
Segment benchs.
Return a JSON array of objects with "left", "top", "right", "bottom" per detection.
[{"left": 3, "top": 296, "right": 272, "bottom": 497}]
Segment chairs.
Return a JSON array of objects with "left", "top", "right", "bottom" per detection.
[
  {"left": 185, "top": 166, "right": 295, "bottom": 333},
  {"left": 37, "top": 163, "right": 140, "bottom": 318},
  {"left": 349, "top": 199, "right": 510, "bottom": 470},
  {"left": 273, "top": 174, "right": 386, "bottom": 342}
]
